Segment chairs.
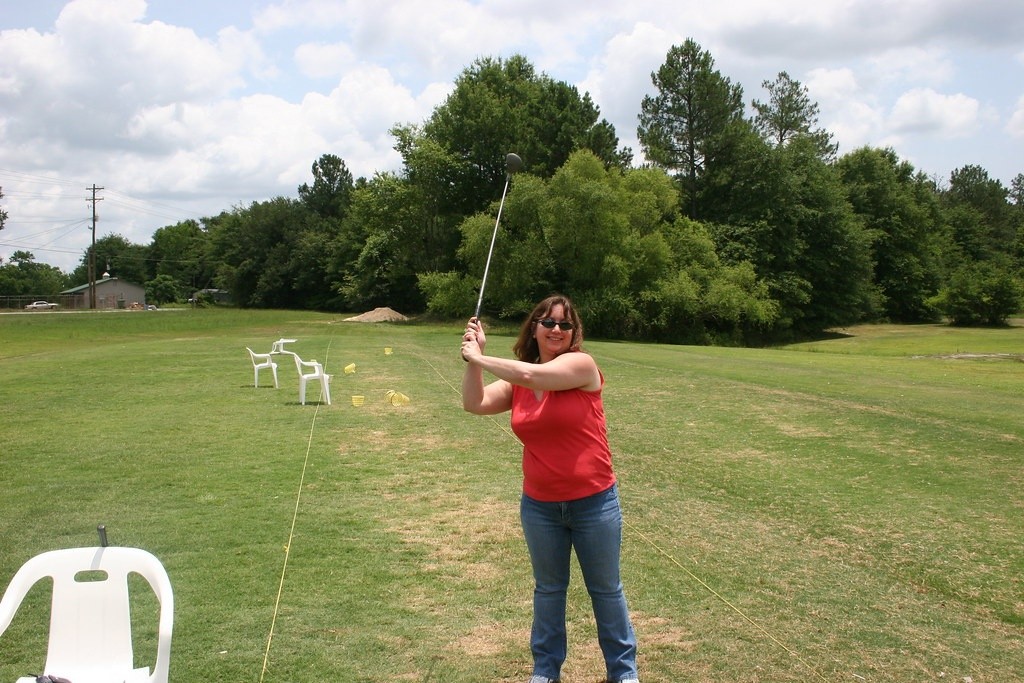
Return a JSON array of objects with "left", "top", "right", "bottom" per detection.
[
  {"left": 269, "top": 337, "right": 298, "bottom": 355},
  {"left": 292, "top": 353, "right": 331, "bottom": 405},
  {"left": 245, "top": 346, "right": 279, "bottom": 389},
  {"left": 0, "top": 546, "right": 174, "bottom": 683}
]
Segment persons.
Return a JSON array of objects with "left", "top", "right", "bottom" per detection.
[{"left": 460, "top": 297, "right": 639, "bottom": 683}]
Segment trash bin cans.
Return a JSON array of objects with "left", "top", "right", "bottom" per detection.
[{"left": 117, "top": 299, "right": 125, "bottom": 309}]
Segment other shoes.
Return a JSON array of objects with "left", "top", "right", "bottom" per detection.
[
  {"left": 617, "top": 678, "right": 639, "bottom": 683},
  {"left": 529, "top": 675, "right": 561, "bottom": 683}
]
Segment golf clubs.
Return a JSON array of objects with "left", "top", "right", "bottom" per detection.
[
  {"left": 96, "top": 523, "right": 110, "bottom": 546},
  {"left": 462, "top": 151, "right": 524, "bottom": 363}
]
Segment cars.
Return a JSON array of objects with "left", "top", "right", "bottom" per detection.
[{"left": 25, "top": 301, "right": 60, "bottom": 310}]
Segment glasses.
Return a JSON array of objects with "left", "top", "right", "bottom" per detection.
[{"left": 536, "top": 319, "right": 574, "bottom": 330}]
